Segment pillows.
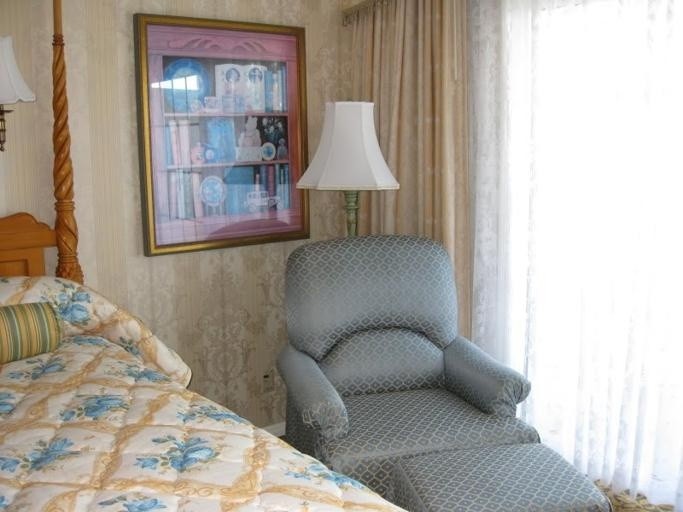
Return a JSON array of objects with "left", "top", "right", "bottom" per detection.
[{"left": 1, "top": 301, "right": 62, "bottom": 365}]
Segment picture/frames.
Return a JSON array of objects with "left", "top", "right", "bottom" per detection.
[{"left": 133, "top": 13, "right": 309, "bottom": 256}]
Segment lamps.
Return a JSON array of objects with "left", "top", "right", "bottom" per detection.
[
  {"left": 1, "top": 35, "right": 35, "bottom": 149},
  {"left": 296, "top": 102, "right": 400, "bottom": 235}
]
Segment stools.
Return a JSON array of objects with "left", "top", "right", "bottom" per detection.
[{"left": 396, "top": 443, "right": 613, "bottom": 512}]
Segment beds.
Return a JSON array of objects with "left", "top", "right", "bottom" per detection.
[{"left": 0, "top": 213, "right": 409, "bottom": 511}]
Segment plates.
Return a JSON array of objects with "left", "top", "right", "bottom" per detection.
[{"left": 163, "top": 57, "right": 211, "bottom": 114}]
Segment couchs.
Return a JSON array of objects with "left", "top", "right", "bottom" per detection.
[{"left": 277, "top": 235, "right": 541, "bottom": 498}]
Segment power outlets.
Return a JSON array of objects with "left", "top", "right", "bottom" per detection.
[{"left": 262, "top": 369, "right": 274, "bottom": 392}]
